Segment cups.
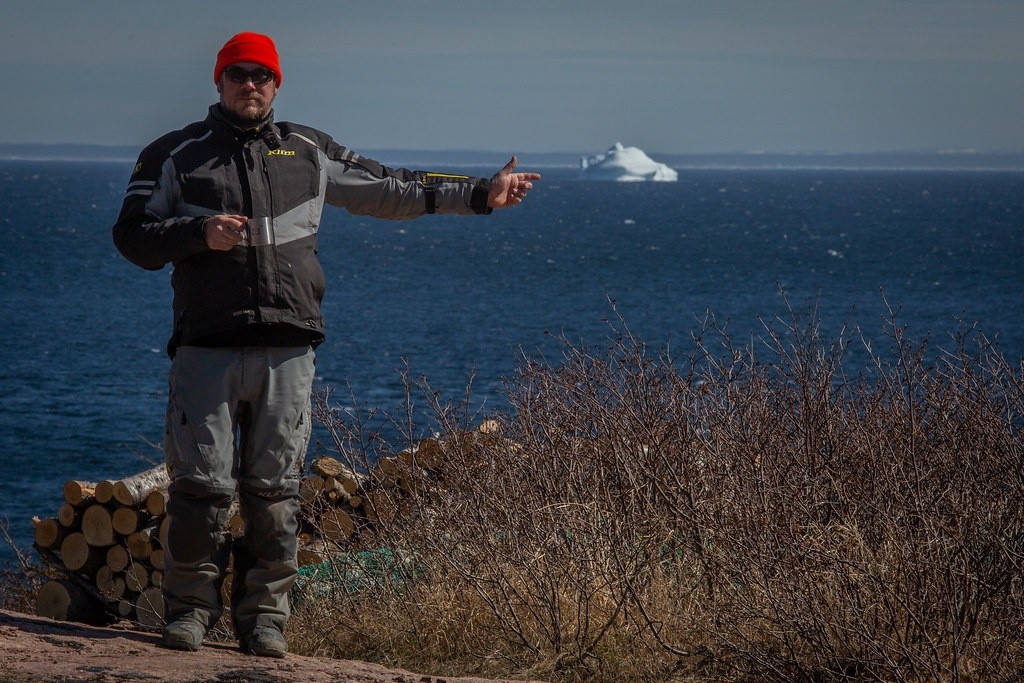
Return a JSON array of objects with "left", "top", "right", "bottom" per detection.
[{"left": 239, "top": 217, "right": 275, "bottom": 247}]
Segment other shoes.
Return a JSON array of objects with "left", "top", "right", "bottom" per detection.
[
  {"left": 163, "top": 621, "right": 203, "bottom": 649},
  {"left": 238, "top": 633, "right": 286, "bottom": 657}
]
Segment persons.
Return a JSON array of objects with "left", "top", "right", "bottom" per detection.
[{"left": 111, "top": 30, "right": 541, "bottom": 656}]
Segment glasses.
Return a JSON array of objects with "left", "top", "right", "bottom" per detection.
[{"left": 221, "top": 65, "right": 278, "bottom": 85}]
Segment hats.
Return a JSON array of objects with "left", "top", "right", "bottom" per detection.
[{"left": 213, "top": 32, "right": 281, "bottom": 89}]
